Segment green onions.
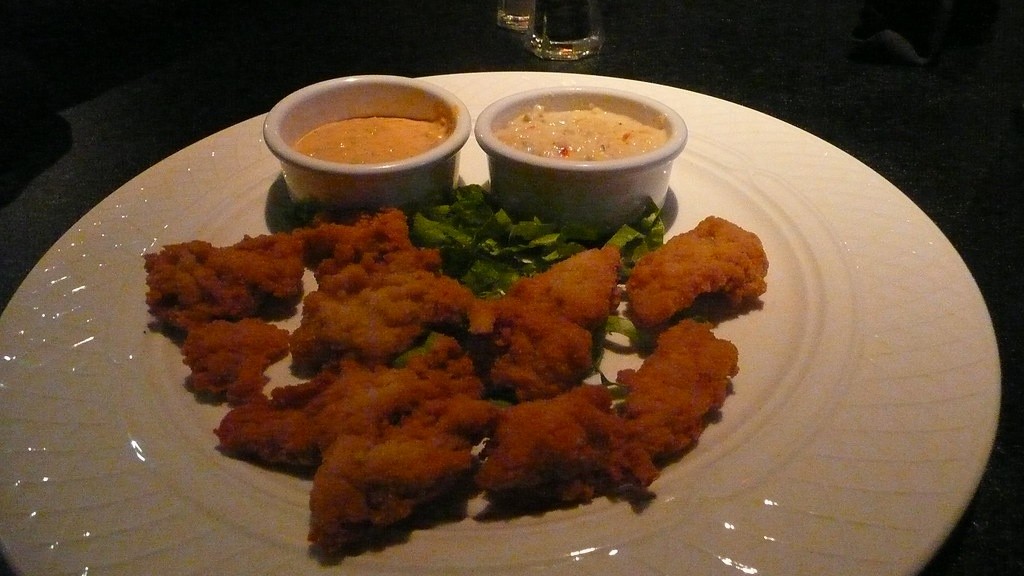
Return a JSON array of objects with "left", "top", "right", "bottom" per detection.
[{"left": 392, "top": 183, "right": 666, "bottom": 406}]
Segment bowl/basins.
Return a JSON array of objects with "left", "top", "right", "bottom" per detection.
[
  {"left": 264, "top": 73, "right": 471, "bottom": 226},
  {"left": 475, "top": 85, "right": 688, "bottom": 237}
]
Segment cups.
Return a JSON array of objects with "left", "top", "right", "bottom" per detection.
[
  {"left": 496, "top": 0, "right": 534, "bottom": 32},
  {"left": 523, "top": 0, "right": 606, "bottom": 61}
]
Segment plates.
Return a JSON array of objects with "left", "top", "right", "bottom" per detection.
[{"left": 0, "top": 70, "right": 1001, "bottom": 576}]
